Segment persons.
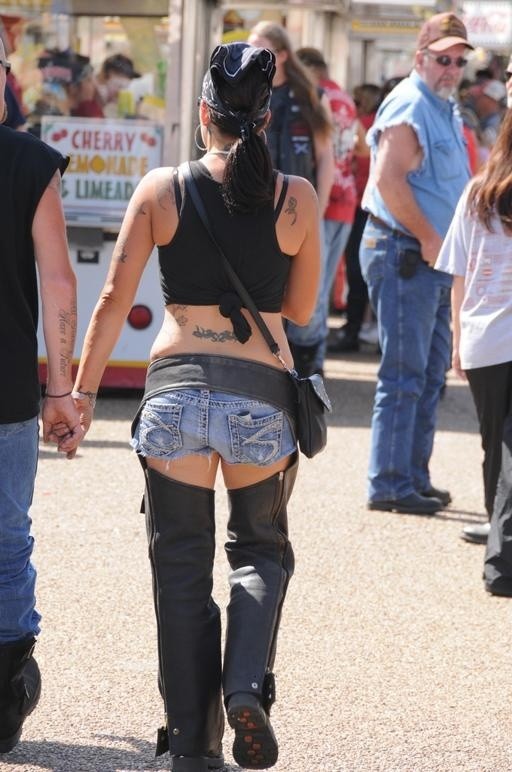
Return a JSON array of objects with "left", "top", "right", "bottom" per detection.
[
  {"left": 0, "top": 28, "right": 89, "bottom": 748},
  {"left": 37, "top": 20, "right": 511, "bottom": 396},
  {"left": 49, "top": 42, "right": 321, "bottom": 766},
  {"left": 352, "top": 11, "right": 475, "bottom": 517},
  {"left": 431, "top": 90, "right": 509, "bottom": 601}
]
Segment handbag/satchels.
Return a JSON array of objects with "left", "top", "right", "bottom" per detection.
[{"left": 296, "top": 373, "right": 332, "bottom": 458}]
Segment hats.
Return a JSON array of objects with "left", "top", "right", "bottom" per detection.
[
  {"left": 417, "top": 13, "right": 474, "bottom": 52},
  {"left": 103, "top": 55, "right": 140, "bottom": 79}
]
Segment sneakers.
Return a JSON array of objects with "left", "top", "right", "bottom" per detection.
[
  {"left": 329, "top": 339, "right": 360, "bottom": 352},
  {"left": 461, "top": 523, "right": 491, "bottom": 544}
]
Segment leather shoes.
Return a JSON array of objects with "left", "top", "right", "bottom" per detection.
[
  {"left": 171, "top": 756, "right": 224, "bottom": 771},
  {"left": 228, "top": 692, "right": 278, "bottom": 770},
  {"left": 421, "top": 487, "right": 451, "bottom": 506},
  {"left": 368, "top": 491, "right": 441, "bottom": 516}
]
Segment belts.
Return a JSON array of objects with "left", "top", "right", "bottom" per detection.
[{"left": 369, "top": 214, "right": 420, "bottom": 243}]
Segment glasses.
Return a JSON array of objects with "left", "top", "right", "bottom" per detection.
[{"left": 425, "top": 55, "right": 467, "bottom": 68}]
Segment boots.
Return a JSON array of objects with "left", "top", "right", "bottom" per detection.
[{"left": 0, "top": 633, "right": 41, "bottom": 752}]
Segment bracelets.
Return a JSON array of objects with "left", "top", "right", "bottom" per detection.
[
  {"left": 71, "top": 384, "right": 101, "bottom": 408},
  {"left": 42, "top": 388, "right": 74, "bottom": 402}
]
